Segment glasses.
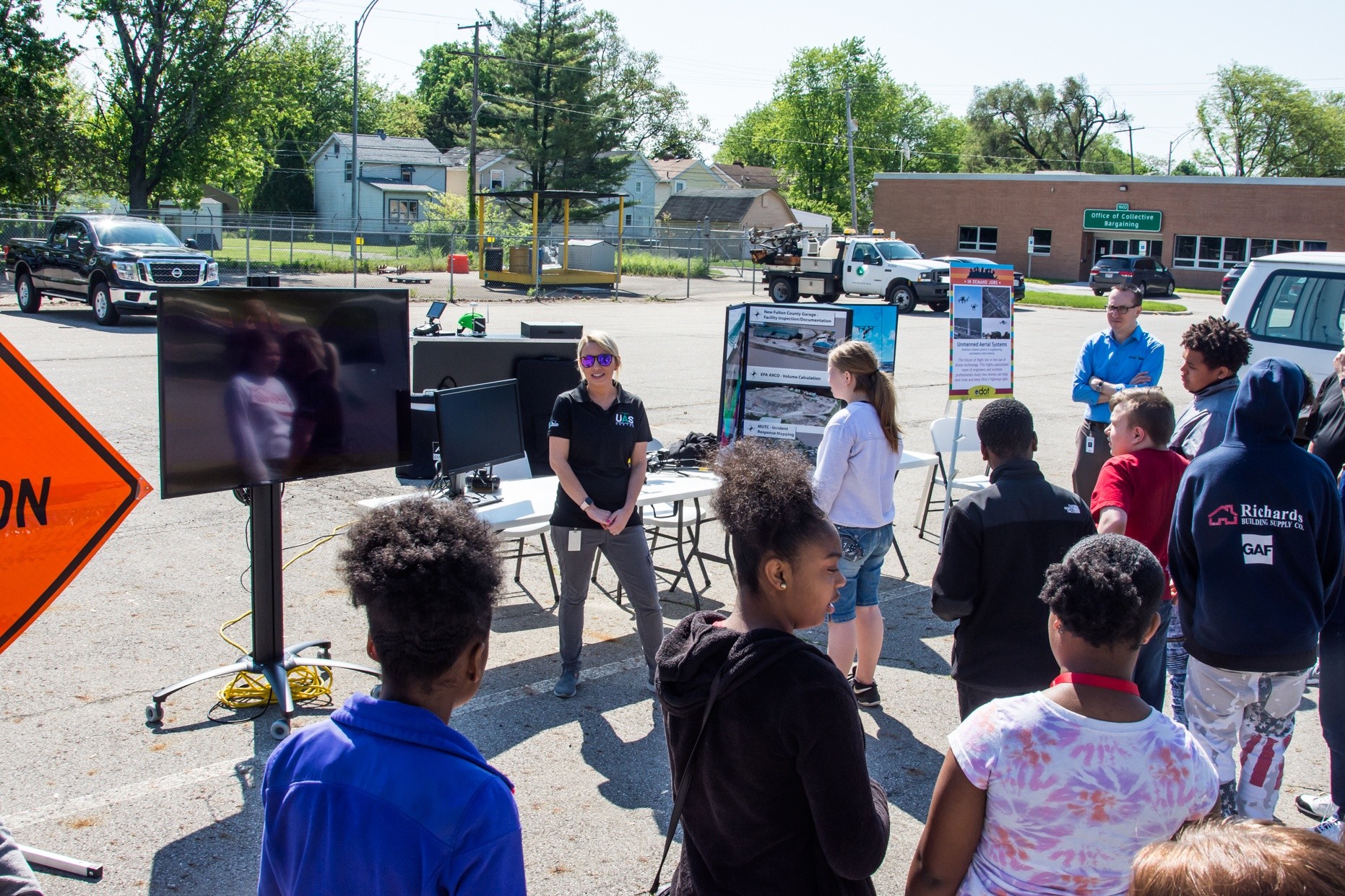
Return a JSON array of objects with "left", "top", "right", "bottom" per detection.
[
  {"left": 579, "top": 353, "right": 613, "bottom": 367},
  {"left": 1104, "top": 305, "right": 1134, "bottom": 314}
]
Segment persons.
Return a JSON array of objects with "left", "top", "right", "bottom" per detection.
[
  {"left": 1068, "top": 281, "right": 1165, "bottom": 503},
  {"left": 213, "top": 299, "right": 347, "bottom": 490},
  {"left": 548, "top": 331, "right": 664, "bottom": 701},
  {"left": 809, "top": 341, "right": 905, "bottom": 710},
  {"left": 1129, "top": 818, "right": 1345, "bottom": 896},
  {"left": 655, "top": 438, "right": 889, "bottom": 896},
  {"left": 904, "top": 538, "right": 1220, "bottom": 895},
  {"left": 930, "top": 398, "right": 1098, "bottom": 726},
  {"left": 1088, "top": 319, "right": 1345, "bottom": 838},
  {"left": 260, "top": 495, "right": 528, "bottom": 896},
  {"left": 0, "top": 820, "right": 44, "bottom": 896}
]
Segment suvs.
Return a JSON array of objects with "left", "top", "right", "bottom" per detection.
[{"left": 1088, "top": 253, "right": 1176, "bottom": 299}]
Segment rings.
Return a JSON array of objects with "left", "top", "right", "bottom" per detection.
[{"left": 616, "top": 531, "right": 619, "bottom": 535}]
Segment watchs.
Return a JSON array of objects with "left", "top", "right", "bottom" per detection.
[
  {"left": 579, "top": 497, "right": 594, "bottom": 511},
  {"left": 1096, "top": 381, "right": 1104, "bottom": 394}
]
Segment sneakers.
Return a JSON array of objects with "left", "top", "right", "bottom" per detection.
[
  {"left": 554, "top": 668, "right": 581, "bottom": 698},
  {"left": 1296, "top": 792, "right": 1340, "bottom": 822},
  {"left": 1308, "top": 813, "right": 1345, "bottom": 846},
  {"left": 854, "top": 666, "right": 882, "bottom": 706},
  {"left": 647, "top": 665, "right": 660, "bottom": 693}
]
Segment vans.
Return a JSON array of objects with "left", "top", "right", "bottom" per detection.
[{"left": 1217, "top": 251, "right": 1345, "bottom": 398}]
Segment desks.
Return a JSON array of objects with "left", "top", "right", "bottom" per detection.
[
  {"left": 357, "top": 472, "right": 720, "bottom": 613},
  {"left": 662, "top": 448, "right": 941, "bottom": 596}
]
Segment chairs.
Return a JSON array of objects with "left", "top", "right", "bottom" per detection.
[
  {"left": 854, "top": 248, "right": 864, "bottom": 260},
  {"left": 591, "top": 438, "right": 711, "bottom": 605},
  {"left": 466, "top": 450, "right": 559, "bottom": 601},
  {"left": 919, "top": 417, "right": 994, "bottom": 539}
]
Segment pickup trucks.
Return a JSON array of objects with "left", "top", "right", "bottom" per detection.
[
  {"left": 2, "top": 214, "right": 221, "bottom": 327},
  {"left": 761, "top": 234, "right": 954, "bottom": 314}
]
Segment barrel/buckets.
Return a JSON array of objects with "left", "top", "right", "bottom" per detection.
[
  {"left": 529, "top": 249, "right": 544, "bottom": 274},
  {"left": 485, "top": 247, "right": 502, "bottom": 287},
  {"left": 509, "top": 246, "right": 529, "bottom": 275}
]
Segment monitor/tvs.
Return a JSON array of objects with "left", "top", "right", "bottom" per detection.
[
  {"left": 156, "top": 286, "right": 410, "bottom": 500},
  {"left": 434, "top": 378, "right": 525, "bottom": 508},
  {"left": 426, "top": 301, "right": 446, "bottom": 324}
]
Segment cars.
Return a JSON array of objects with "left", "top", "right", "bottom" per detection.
[
  {"left": 925, "top": 256, "right": 1025, "bottom": 303},
  {"left": 1289, "top": 277, "right": 1309, "bottom": 308},
  {"left": 1220, "top": 262, "right": 1293, "bottom": 306}
]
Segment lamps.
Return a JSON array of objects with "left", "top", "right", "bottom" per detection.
[
  {"left": 323, "top": 154, "right": 338, "bottom": 160},
  {"left": 1119, "top": 185, "right": 1128, "bottom": 192}
]
